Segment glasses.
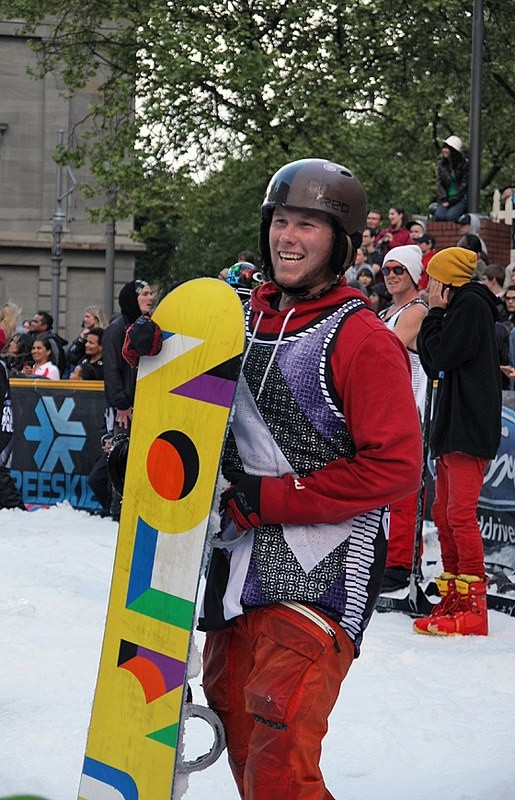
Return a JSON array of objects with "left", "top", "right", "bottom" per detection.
[
  {"left": 504, "top": 296, "right": 515, "bottom": 302},
  {"left": 381, "top": 266, "right": 407, "bottom": 276}
]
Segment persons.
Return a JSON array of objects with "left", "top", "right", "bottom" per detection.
[
  {"left": 412, "top": 244, "right": 503, "bottom": 639},
  {"left": 434, "top": 134, "right": 469, "bottom": 225},
  {"left": 121, "top": 157, "right": 431, "bottom": 800},
  {"left": 0, "top": 202, "right": 515, "bottom": 514}
]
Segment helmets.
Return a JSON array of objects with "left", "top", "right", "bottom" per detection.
[
  {"left": 225, "top": 261, "right": 264, "bottom": 297},
  {"left": 262, "top": 157, "right": 369, "bottom": 272}
]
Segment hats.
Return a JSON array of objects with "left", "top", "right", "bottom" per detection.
[
  {"left": 135, "top": 280, "right": 149, "bottom": 297},
  {"left": 438, "top": 136, "right": 463, "bottom": 155},
  {"left": 412, "top": 219, "right": 427, "bottom": 231},
  {"left": 426, "top": 247, "right": 478, "bottom": 288},
  {"left": 355, "top": 264, "right": 375, "bottom": 284},
  {"left": 413, "top": 233, "right": 435, "bottom": 244},
  {"left": 452, "top": 214, "right": 470, "bottom": 226},
  {"left": 382, "top": 245, "right": 424, "bottom": 286},
  {"left": 365, "top": 283, "right": 393, "bottom": 299}
]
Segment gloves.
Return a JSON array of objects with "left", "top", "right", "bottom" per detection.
[
  {"left": 221, "top": 467, "right": 262, "bottom": 532},
  {"left": 130, "top": 315, "right": 162, "bottom": 356}
]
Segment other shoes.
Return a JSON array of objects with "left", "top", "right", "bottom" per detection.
[{"left": 375, "top": 567, "right": 408, "bottom": 594}]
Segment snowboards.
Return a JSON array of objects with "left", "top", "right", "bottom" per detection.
[{"left": 75, "top": 276, "right": 247, "bottom": 800}]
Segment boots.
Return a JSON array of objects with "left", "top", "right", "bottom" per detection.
[
  {"left": 413, "top": 572, "right": 458, "bottom": 635},
  {"left": 427, "top": 574, "right": 491, "bottom": 637}
]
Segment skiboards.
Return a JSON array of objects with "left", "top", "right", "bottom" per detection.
[{"left": 376, "top": 579, "right": 515, "bottom": 628}]
